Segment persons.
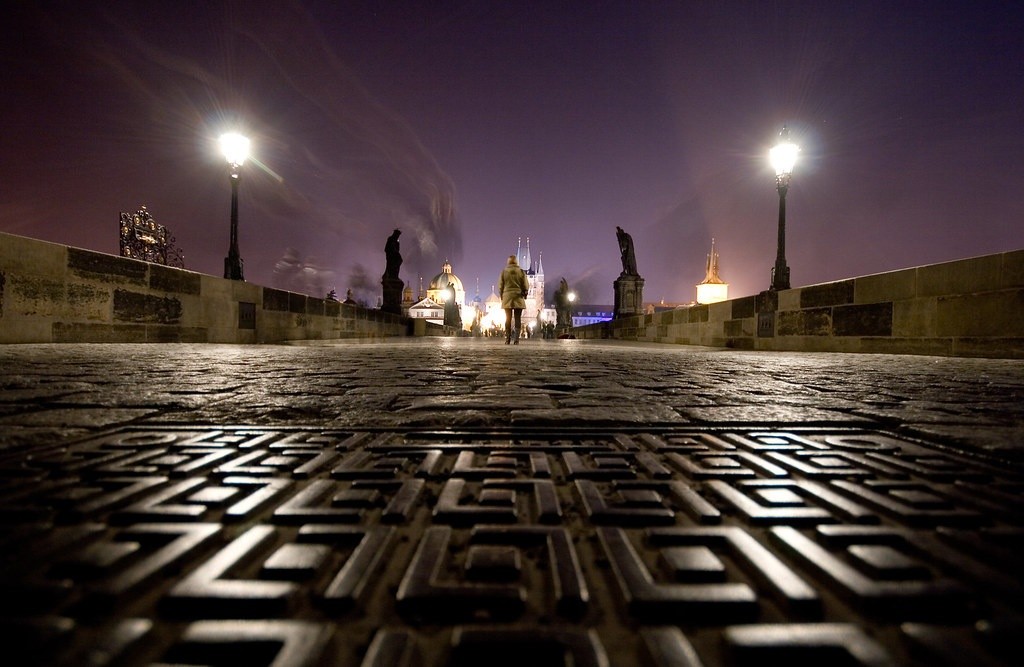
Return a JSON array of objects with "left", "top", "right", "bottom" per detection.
[
  {"left": 382, "top": 229, "right": 403, "bottom": 281},
  {"left": 615, "top": 225, "right": 640, "bottom": 277},
  {"left": 542, "top": 320, "right": 554, "bottom": 340},
  {"left": 499, "top": 255, "right": 529, "bottom": 345}
]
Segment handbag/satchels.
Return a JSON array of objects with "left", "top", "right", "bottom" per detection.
[{"left": 521, "top": 279, "right": 528, "bottom": 299}]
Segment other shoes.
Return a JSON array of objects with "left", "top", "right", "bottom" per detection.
[
  {"left": 504, "top": 332, "right": 510, "bottom": 344},
  {"left": 514, "top": 332, "right": 519, "bottom": 345}
]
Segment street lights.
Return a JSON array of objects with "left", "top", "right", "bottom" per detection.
[
  {"left": 767, "top": 136, "right": 803, "bottom": 292},
  {"left": 219, "top": 131, "right": 252, "bottom": 283},
  {"left": 566, "top": 292, "right": 577, "bottom": 327}
]
